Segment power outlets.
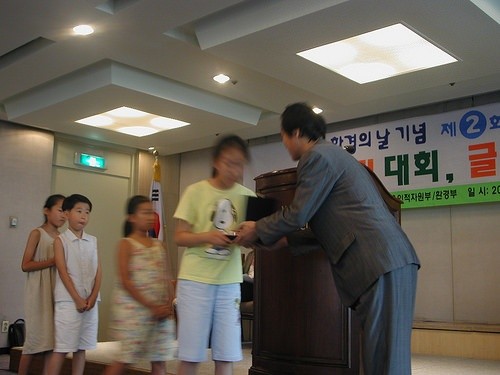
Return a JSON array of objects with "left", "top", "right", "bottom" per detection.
[{"left": 2, "top": 320, "right": 9, "bottom": 333}]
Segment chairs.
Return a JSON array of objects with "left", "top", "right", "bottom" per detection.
[{"left": 240, "top": 301, "right": 254, "bottom": 348}]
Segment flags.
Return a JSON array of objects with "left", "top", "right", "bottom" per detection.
[{"left": 147, "top": 161, "right": 168, "bottom": 242}]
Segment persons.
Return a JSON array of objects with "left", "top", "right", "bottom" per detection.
[
  {"left": 106, "top": 195, "right": 176, "bottom": 375},
  {"left": 45, "top": 194, "right": 102, "bottom": 375},
  {"left": 18, "top": 194, "right": 68, "bottom": 375},
  {"left": 173, "top": 134, "right": 259, "bottom": 375},
  {"left": 232, "top": 102, "right": 421, "bottom": 375}
]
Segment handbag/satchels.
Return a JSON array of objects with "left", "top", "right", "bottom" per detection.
[{"left": 8, "top": 318, "right": 25, "bottom": 347}]
